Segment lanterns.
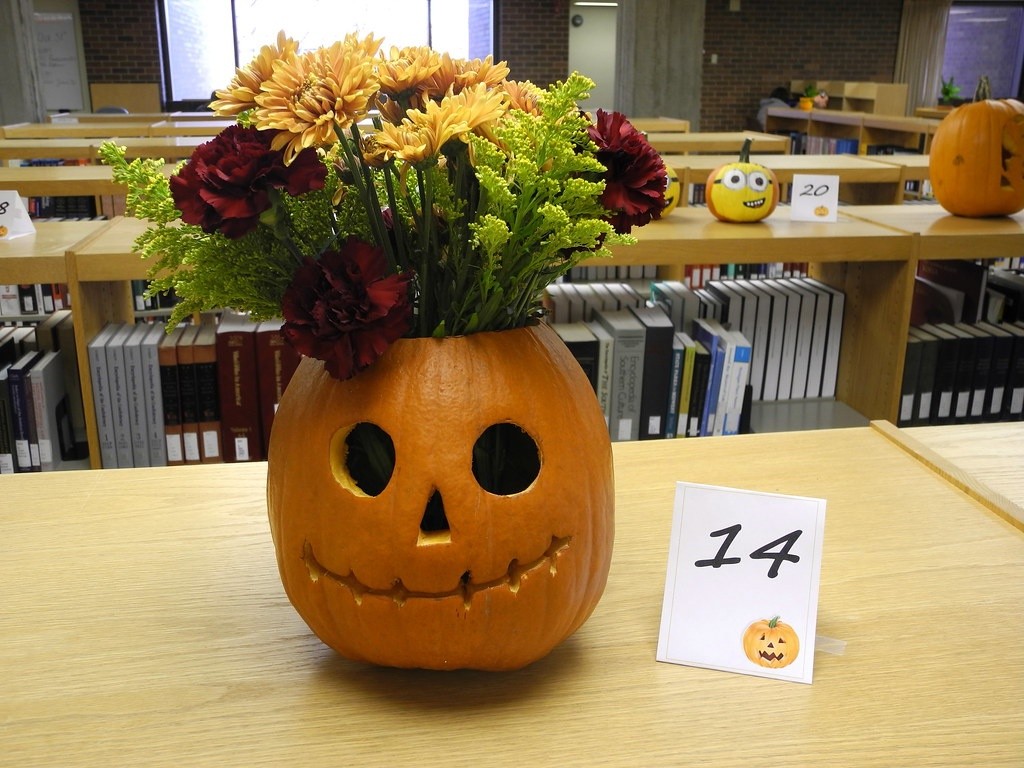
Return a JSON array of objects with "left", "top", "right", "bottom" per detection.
[
  {"left": 928, "top": 75, "right": 1024, "bottom": 219},
  {"left": 0, "top": 225, "right": 9, "bottom": 237},
  {"left": 703, "top": 135, "right": 778, "bottom": 223},
  {"left": 265, "top": 316, "right": 618, "bottom": 675}
]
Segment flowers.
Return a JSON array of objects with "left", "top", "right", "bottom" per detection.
[{"left": 97, "top": 28, "right": 666, "bottom": 381}]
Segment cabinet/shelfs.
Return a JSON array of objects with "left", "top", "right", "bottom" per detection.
[{"left": 0, "top": 79, "right": 1024, "bottom": 768}]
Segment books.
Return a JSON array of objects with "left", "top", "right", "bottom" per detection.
[
  {"left": 17, "top": 196, "right": 132, "bottom": 223},
  {"left": 687, "top": 183, "right": 706, "bottom": 204},
  {"left": 15, "top": 159, "right": 89, "bottom": 168},
  {"left": 778, "top": 179, "right": 791, "bottom": 202},
  {"left": 214, "top": 304, "right": 260, "bottom": 464},
  {"left": 0, "top": 280, "right": 220, "bottom": 472},
  {"left": 542, "top": 258, "right": 848, "bottom": 444},
  {"left": 774, "top": 128, "right": 921, "bottom": 158},
  {"left": 897, "top": 258, "right": 1023, "bottom": 431},
  {"left": 903, "top": 178, "right": 935, "bottom": 205},
  {"left": 258, "top": 313, "right": 300, "bottom": 463}
]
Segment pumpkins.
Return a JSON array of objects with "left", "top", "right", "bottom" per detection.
[
  {"left": 705, "top": 138, "right": 778, "bottom": 222},
  {"left": 640, "top": 131, "right": 680, "bottom": 219}
]
